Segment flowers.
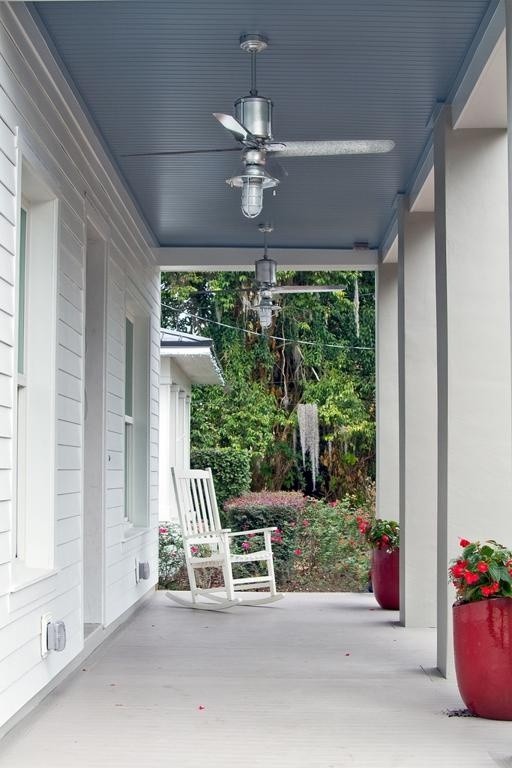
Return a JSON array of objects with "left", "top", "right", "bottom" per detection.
[
  {"left": 445, "top": 537, "right": 512, "bottom": 605},
  {"left": 355, "top": 516, "right": 399, "bottom": 554}
]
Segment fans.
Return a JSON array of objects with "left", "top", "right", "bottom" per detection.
[
  {"left": 212, "top": 34, "right": 397, "bottom": 157},
  {"left": 195, "top": 221, "right": 347, "bottom": 294}
]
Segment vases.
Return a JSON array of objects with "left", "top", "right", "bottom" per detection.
[
  {"left": 451, "top": 596, "right": 512, "bottom": 720},
  {"left": 369, "top": 549, "right": 400, "bottom": 610}
]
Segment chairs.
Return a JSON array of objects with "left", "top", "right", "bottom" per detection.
[{"left": 164, "top": 466, "right": 285, "bottom": 611}]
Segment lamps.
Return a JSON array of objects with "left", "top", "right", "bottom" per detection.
[
  {"left": 253, "top": 298, "right": 282, "bottom": 329},
  {"left": 226, "top": 147, "right": 282, "bottom": 222}
]
